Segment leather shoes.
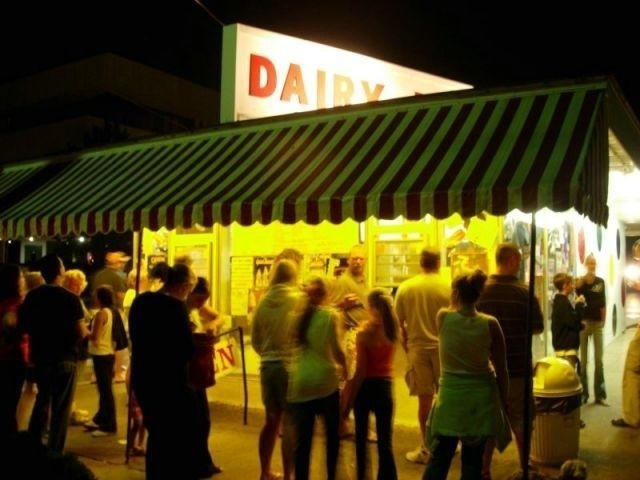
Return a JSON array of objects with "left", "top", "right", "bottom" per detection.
[{"left": 611, "top": 418, "right": 629, "bottom": 427}]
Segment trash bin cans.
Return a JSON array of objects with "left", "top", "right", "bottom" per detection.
[{"left": 528, "top": 357, "right": 583, "bottom": 465}]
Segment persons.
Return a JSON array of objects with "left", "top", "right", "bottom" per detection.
[
  {"left": 341, "top": 289, "right": 397, "bottom": 480},
  {"left": 251, "top": 259, "right": 307, "bottom": 479},
  {"left": 282, "top": 279, "right": 349, "bottom": 480},
  {"left": 129, "top": 261, "right": 199, "bottom": 480},
  {"left": 476, "top": 245, "right": 544, "bottom": 480},
  {"left": 421, "top": 268, "right": 512, "bottom": 480},
  {"left": 394, "top": 248, "right": 460, "bottom": 464},
  {"left": 1, "top": 250, "right": 639, "bottom": 457}
]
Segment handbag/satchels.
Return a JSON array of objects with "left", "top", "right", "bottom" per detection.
[
  {"left": 191, "top": 332, "right": 215, "bottom": 388},
  {"left": 113, "top": 307, "right": 129, "bottom": 351}
]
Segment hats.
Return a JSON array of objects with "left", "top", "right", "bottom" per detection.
[{"left": 106, "top": 252, "right": 130, "bottom": 264}]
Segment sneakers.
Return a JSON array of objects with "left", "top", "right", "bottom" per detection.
[
  {"left": 72, "top": 409, "right": 108, "bottom": 436},
  {"left": 406, "top": 446, "right": 431, "bottom": 464}
]
